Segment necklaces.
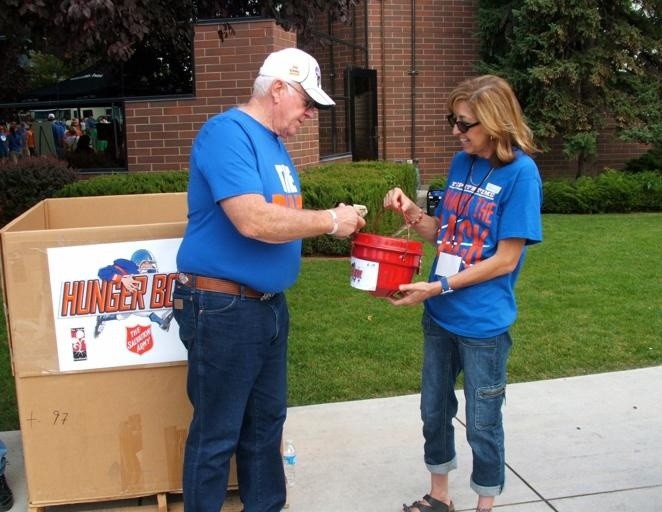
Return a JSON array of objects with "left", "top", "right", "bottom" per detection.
[{"left": 469, "top": 157, "right": 495, "bottom": 189}]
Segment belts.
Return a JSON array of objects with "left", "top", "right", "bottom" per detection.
[{"left": 175, "top": 273, "right": 276, "bottom": 302}]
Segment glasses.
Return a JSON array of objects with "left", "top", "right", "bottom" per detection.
[
  {"left": 447, "top": 113, "right": 480, "bottom": 133},
  {"left": 286, "top": 81, "right": 313, "bottom": 109}
]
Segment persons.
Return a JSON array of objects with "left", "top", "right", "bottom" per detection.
[
  {"left": 169, "top": 45, "right": 369, "bottom": 512},
  {"left": 383, "top": 74, "right": 545, "bottom": 512},
  {"left": 2, "top": 111, "right": 124, "bottom": 169}
]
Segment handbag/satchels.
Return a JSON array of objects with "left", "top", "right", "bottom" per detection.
[{"left": 23, "top": 149, "right": 31, "bottom": 156}]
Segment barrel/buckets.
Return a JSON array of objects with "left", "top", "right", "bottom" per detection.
[{"left": 350, "top": 202, "right": 423, "bottom": 297}]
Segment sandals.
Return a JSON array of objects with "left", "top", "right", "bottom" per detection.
[{"left": 403, "top": 495, "right": 455, "bottom": 512}]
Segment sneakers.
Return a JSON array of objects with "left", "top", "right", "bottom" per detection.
[
  {"left": 159, "top": 312, "right": 173, "bottom": 330},
  {"left": 95, "top": 316, "right": 105, "bottom": 338},
  {"left": 0, "top": 474, "right": 13, "bottom": 512}
]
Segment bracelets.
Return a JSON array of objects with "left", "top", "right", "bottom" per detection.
[
  {"left": 439, "top": 276, "right": 455, "bottom": 298},
  {"left": 406, "top": 207, "right": 426, "bottom": 227},
  {"left": 325, "top": 209, "right": 341, "bottom": 235}
]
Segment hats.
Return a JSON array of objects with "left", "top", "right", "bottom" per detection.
[
  {"left": 258, "top": 48, "right": 336, "bottom": 110},
  {"left": 48, "top": 113, "right": 55, "bottom": 119}
]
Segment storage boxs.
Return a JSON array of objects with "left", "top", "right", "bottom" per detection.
[{"left": 1, "top": 193, "right": 288, "bottom": 509}]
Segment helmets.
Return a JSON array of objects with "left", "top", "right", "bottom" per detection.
[{"left": 131, "top": 249, "right": 159, "bottom": 273}]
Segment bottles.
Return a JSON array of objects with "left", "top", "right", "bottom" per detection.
[{"left": 284, "top": 438, "right": 298, "bottom": 486}]
